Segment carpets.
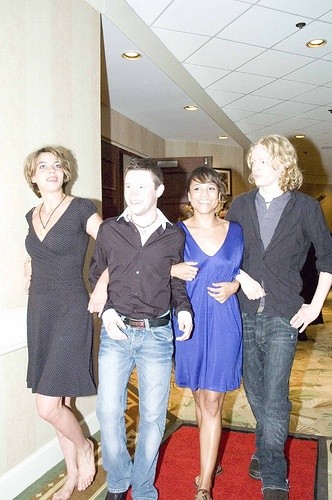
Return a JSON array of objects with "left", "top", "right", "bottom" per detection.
[{"left": 121, "top": 424, "right": 320, "bottom": 500}]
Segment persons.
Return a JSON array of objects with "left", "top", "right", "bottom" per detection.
[
  {"left": 222, "top": 135, "right": 332, "bottom": 500},
  {"left": 170, "top": 167, "right": 245, "bottom": 500},
  {"left": 89, "top": 157, "right": 195, "bottom": 500},
  {"left": 297, "top": 243, "right": 324, "bottom": 340},
  {"left": 19, "top": 146, "right": 112, "bottom": 500}
]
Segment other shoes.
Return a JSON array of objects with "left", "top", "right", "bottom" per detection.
[
  {"left": 249, "top": 459, "right": 261, "bottom": 479},
  {"left": 196, "top": 489, "right": 212, "bottom": 500},
  {"left": 194, "top": 465, "right": 223, "bottom": 488},
  {"left": 263, "top": 488, "right": 288, "bottom": 500},
  {"left": 105, "top": 491, "right": 127, "bottom": 500}
]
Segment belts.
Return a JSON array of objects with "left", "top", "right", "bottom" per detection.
[{"left": 117, "top": 313, "right": 170, "bottom": 327}]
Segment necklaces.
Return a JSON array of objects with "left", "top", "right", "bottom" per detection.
[{"left": 37, "top": 191, "right": 68, "bottom": 230}]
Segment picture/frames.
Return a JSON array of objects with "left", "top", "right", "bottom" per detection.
[{"left": 214, "top": 167, "right": 232, "bottom": 195}]
